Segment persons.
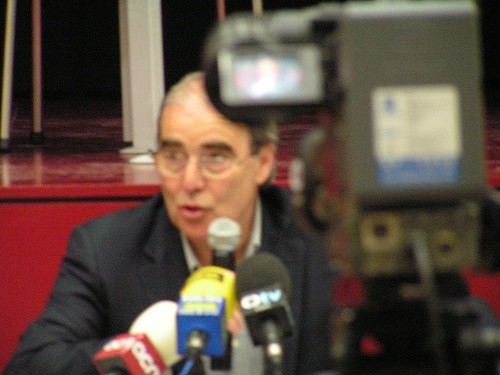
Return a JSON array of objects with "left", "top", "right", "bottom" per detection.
[{"left": 0, "top": 71, "right": 335, "bottom": 375}]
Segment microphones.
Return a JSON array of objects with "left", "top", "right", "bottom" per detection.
[{"left": 92, "top": 219, "right": 295, "bottom": 375}]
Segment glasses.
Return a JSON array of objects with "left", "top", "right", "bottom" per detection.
[{"left": 153, "top": 144, "right": 263, "bottom": 177}]
